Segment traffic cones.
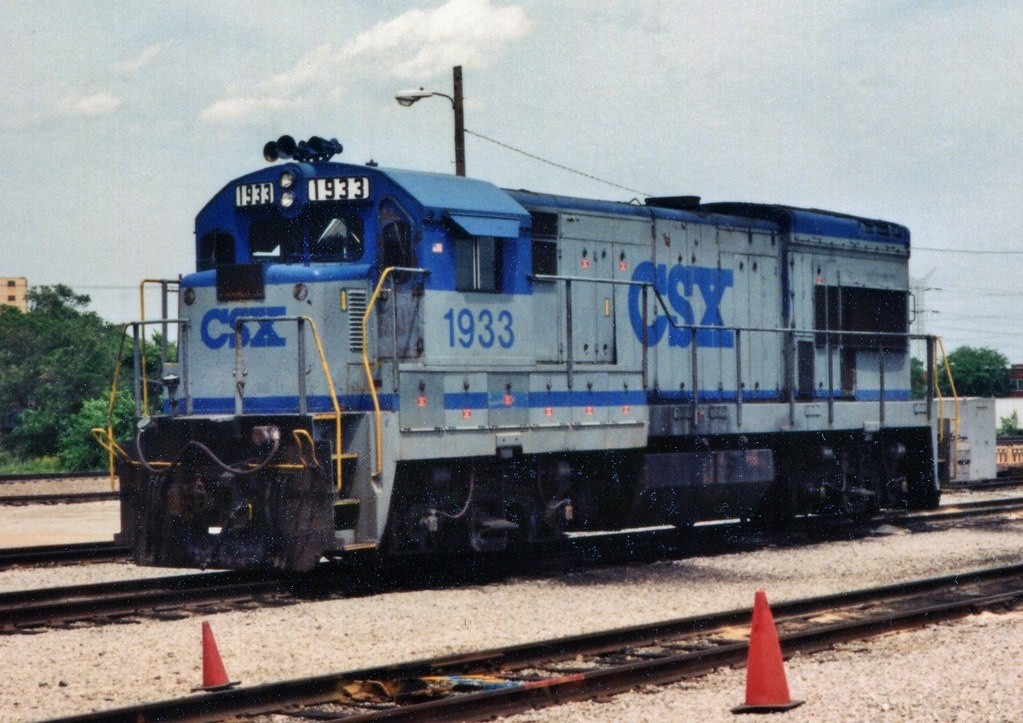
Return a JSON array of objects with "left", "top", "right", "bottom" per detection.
[
  {"left": 729, "top": 592, "right": 807, "bottom": 715},
  {"left": 190, "top": 620, "right": 242, "bottom": 692}
]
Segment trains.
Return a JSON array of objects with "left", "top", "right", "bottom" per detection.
[{"left": 112, "top": 134, "right": 944, "bottom": 597}]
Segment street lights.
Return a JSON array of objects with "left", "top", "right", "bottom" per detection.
[{"left": 396, "top": 85, "right": 468, "bottom": 176}]
